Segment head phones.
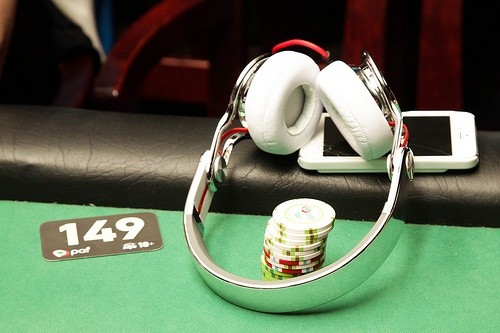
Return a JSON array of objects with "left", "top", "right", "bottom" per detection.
[{"left": 183, "top": 38, "right": 417, "bottom": 317}]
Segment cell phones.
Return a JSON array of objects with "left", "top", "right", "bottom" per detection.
[{"left": 295, "top": 106, "right": 480, "bottom": 174}]
